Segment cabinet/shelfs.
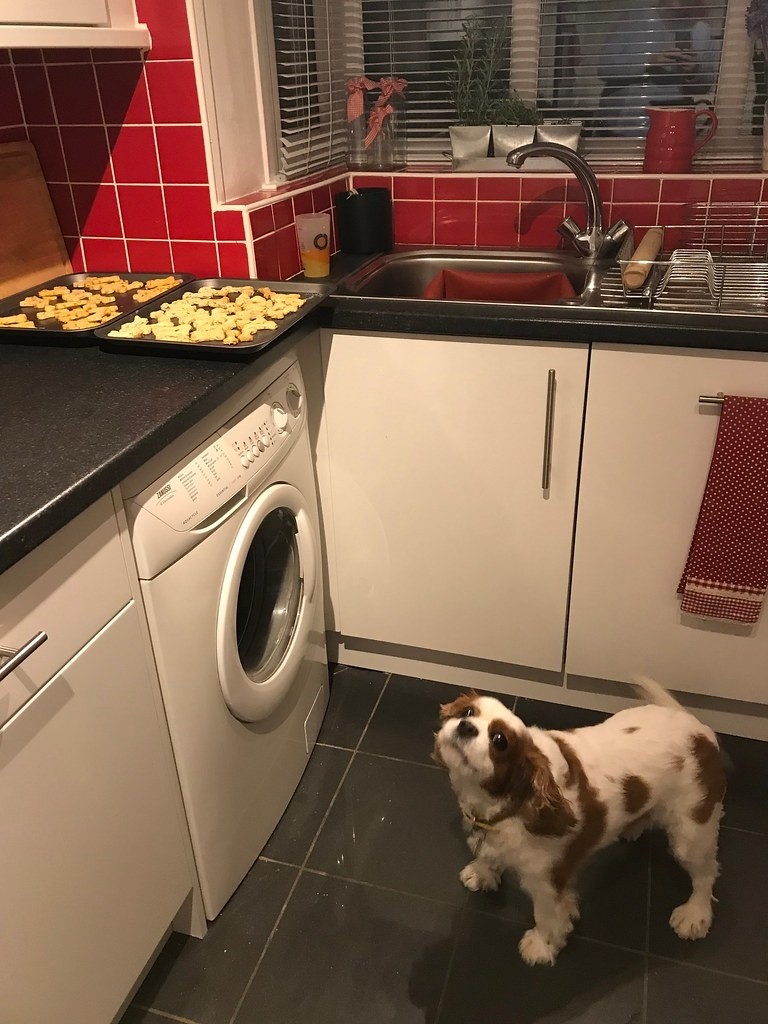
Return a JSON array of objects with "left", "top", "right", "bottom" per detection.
[
  {"left": 296, "top": 296, "right": 768, "bottom": 745},
  {"left": 0, "top": 459, "right": 207, "bottom": 1024},
  {"left": 0, "top": 0, "right": 154, "bottom": 53}
]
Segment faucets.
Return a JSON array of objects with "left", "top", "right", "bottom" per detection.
[{"left": 504, "top": 139, "right": 608, "bottom": 265}]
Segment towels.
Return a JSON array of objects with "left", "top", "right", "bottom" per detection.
[{"left": 674, "top": 394, "right": 768, "bottom": 636}]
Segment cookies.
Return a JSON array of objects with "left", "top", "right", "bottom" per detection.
[{"left": 0, "top": 276, "right": 307, "bottom": 346}]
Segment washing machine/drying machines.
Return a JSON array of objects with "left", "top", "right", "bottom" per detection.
[{"left": 117, "top": 347, "right": 339, "bottom": 935}]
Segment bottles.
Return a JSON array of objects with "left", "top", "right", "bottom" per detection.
[
  {"left": 374, "top": 75, "right": 408, "bottom": 170},
  {"left": 344, "top": 76, "right": 372, "bottom": 170}
]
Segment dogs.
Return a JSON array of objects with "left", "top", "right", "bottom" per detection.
[{"left": 429, "top": 676, "right": 729, "bottom": 967}]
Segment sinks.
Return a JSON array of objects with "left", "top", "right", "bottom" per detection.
[{"left": 327, "top": 244, "right": 619, "bottom": 311}]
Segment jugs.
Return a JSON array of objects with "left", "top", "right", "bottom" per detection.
[{"left": 641, "top": 107, "right": 718, "bottom": 174}]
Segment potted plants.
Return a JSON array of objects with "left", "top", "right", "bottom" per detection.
[
  {"left": 485, "top": 87, "right": 537, "bottom": 157},
  {"left": 442, "top": 11, "right": 513, "bottom": 157},
  {"left": 537, "top": 116, "right": 583, "bottom": 155}
]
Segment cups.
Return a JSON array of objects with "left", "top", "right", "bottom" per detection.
[{"left": 295, "top": 212, "right": 333, "bottom": 278}]
